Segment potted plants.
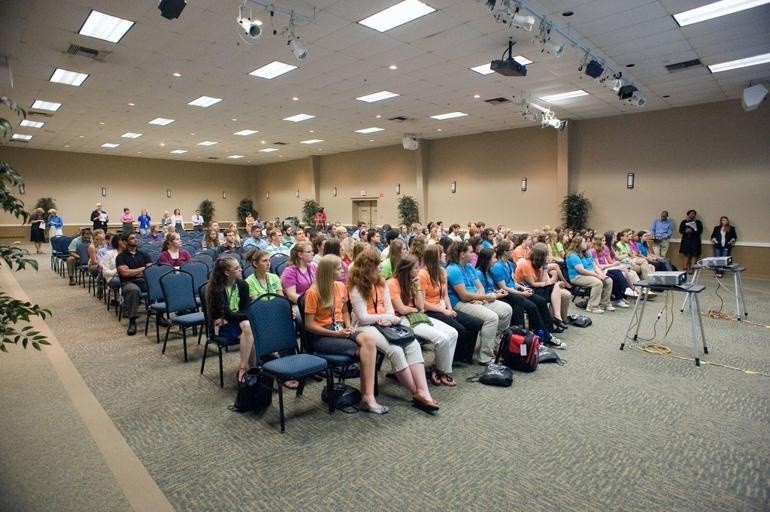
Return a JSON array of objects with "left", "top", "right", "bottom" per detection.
[{"left": 35, "top": 197, "right": 58, "bottom": 243}]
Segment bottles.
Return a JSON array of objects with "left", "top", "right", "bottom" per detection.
[{"left": 536, "top": 327, "right": 545, "bottom": 347}]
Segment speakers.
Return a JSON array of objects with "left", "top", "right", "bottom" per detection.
[
  {"left": 740, "top": 83, "right": 768, "bottom": 113},
  {"left": 401, "top": 135, "right": 419, "bottom": 151}
]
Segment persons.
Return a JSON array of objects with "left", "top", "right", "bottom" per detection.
[
  {"left": 47, "top": 208, "right": 64, "bottom": 253},
  {"left": 68, "top": 203, "right": 676, "bottom": 417},
  {"left": 711, "top": 215, "right": 738, "bottom": 278},
  {"left": 678, "top": 210, "right": 703, "bottom": 274},
  {"left": 28, "top": 207, "right": 47, "bottom": 256}
]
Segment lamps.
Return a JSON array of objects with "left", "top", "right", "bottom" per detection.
[
  {"left": 235, "top": 1, "right": 318, "bottom": 61},
  {"left": 483, "top": 0, "right": 645, "bottom": 108},
  {"left": 102, "top": 187, "right": 106, "bottom": 197},
  {"left": 333, "top": 187, "right": 337, "bottom": 196},
  {"left": 511, "top": 94, "right": 567, "bottom": 132},
  {"left": 167, "top": 189, "right": 172, "bottom": 198},
  {"left": 296, "top": 190, "right": 299, "bottom": 198},
  {"left": 19, "top": 183, "right": 25, "bottom": 194},
  {"left": 521, "top": 177, "right": 527, "bottom": 192},
  {"left": 395, "top": 184, "right": 400, "bottom": 195},
  {"left": 450, "top": 180, "right": 456, "bottom": 193},
  {"left": 223, "top": 192, "right": 226, "bottom": 199},
  {"left": 627, "top": 172, "right": 634, "bottom": 189},
  {"left": 266, "top": 191, "right": 269, "bottom": 198}
]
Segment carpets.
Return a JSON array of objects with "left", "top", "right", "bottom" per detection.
[
  {"left": 0, "top": 275, "right": 134, "bottom": 512},
  {"left": 0, "top": 230, "right": 770, "bottom": 512}
]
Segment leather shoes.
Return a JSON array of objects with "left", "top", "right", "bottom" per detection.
[
  {"left": 412, "top": 391, "right": 440, "bottom": 410},
  {"left": 688, "top": 271, "right": 694, "bottom": 275},
  {"left": 155, "top": 316, "right": 171, "bottom": 327},
  {"left": 359, "top": 402, "right": 389, "bottom": 414},
  {"left": 126, "top": 322, "right": 138, "bottom": 336}
]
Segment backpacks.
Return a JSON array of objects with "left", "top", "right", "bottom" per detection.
[
  {"left": 572, "top": 286, "right": 591, "bottom": 296},
  {"left": 494, "top": 325, "right": 540, "bottom": 374}
]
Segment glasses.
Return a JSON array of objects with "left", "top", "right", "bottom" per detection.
[{"left": 506, "top": 248, "right": 514, "bottom": 252}]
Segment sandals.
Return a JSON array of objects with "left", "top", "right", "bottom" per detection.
[
  {"left": 440, "top": 372, "right": 456, "bottom": 387},
  {"left": 429, "top": 368, "right": 441, "bottom": 386}
]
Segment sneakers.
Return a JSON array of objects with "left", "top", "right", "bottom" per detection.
[
  {"left": 715, "top": 269, "right": 724, "bottom": 278},
  {"left": 613, "top": 298, "right": 631, "bottom": 308},
  {"left": 69, "top": 277, "right": 77, "bottom": 286},
  {"left": 599, "top": 303, "right": 616, "bottom": 311},
  {"left": 545, "top": 332, "right": 567, "bottom": 349},
  {"left": 624, "top": 287, "right": 640, "bottom": 297},
  {"left": 641, "top": 290, "right": 658, "bottom": 299},
  {"left": 585, "top": 303, "right": 605, "bottom": 314},
  {"left": 236, "top": 369, "right": 247, "bottom": 388},
  {"left": 548, "top": 316, "right": 568, "bottom": 333}
]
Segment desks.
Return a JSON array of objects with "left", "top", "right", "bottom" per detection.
[
  {"left": 620, "top": 279, "right": 708, "bottom": 367},
  {"left": 681, "top": 264, "right": 748, "bottom": 322}
]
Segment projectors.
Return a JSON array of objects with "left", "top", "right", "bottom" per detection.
[
  {"left": 646, "top": 271, "right": 687, "bottom": 286},
  {"left": 702, "top": 256, "right": 732, "bottom": 267},
  {"left": 489, "top": 60, "right": 526, "bottom": 77}
]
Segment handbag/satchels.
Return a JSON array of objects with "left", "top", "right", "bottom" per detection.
[
  {"left": 372, "top": 322, "right": 416, "bottom": 345},
  {"left": 320, "top": 382, "right": 363, "bottom": 415},
  {"left": 537, "top": 343, "right": 567, "bottom": 367},
  {"left": 227, "top": 366, "right": 275, "bottom": 417},
  {"left": 479, "top": 363, "right": 513, "bottom": 388},
  {"left": 574, "top": 300, "right": 588, "bottom": 309},
  {"left": 333, "top": 361, "right": 361, "bottom": 379},
  {"left": 568, "top": 313, "right": 592, "bottom": 328}
]
